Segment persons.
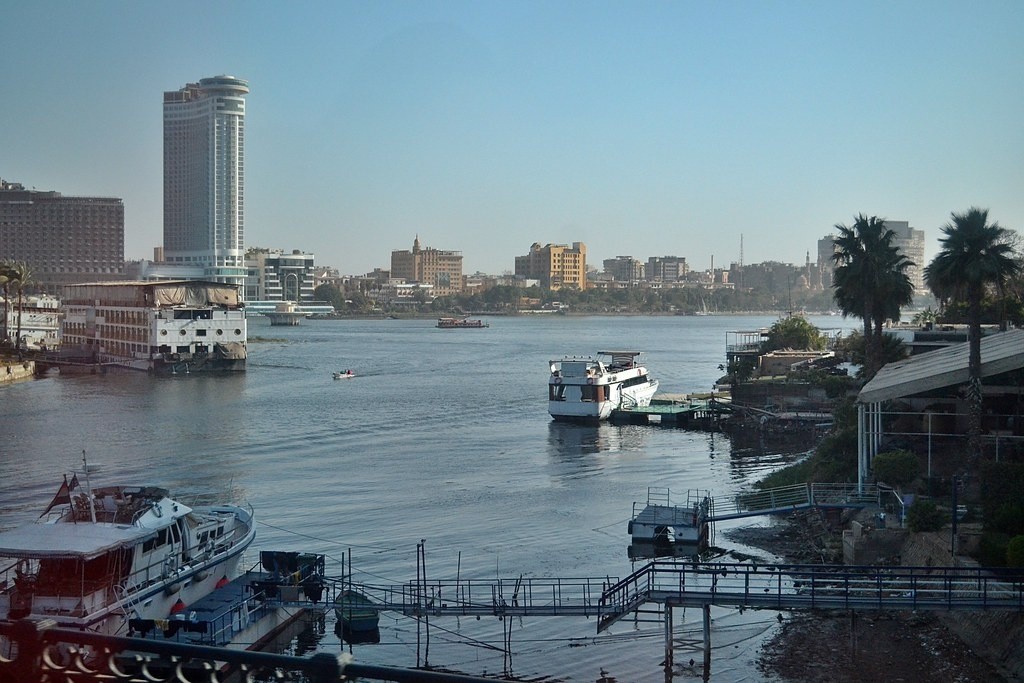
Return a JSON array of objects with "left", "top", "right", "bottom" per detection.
[
  {"left": 73, "top": 492, "right": 88, "bottom": 509},
  {"left": 340, "top": 369, "right": 353, "bottom": 375},
  {"left": 880, "top": 510, "right": 887, "bottom": 521}
]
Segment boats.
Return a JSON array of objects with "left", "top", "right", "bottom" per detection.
[
  {"left": 547, "top": 350, "right": 659, "bottom": 421},
  {"left": 334, "top": 590, "right": 380, "bottom": 629},
  {"left": 333, "top": 372, "right": 355, "bottom": 380},
  {"left": 435, "top": 317, "right": 486, "bottom": 328},
  {"left": 0, "top": 448, "right": 257, "bottom": 683}
]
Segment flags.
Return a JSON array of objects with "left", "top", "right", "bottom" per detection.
[
  {"left": 68, "top": 476, "right": 79, "bottom": 492},
  {"left": 39, "top": 479, "right": 71, "bottom": 519}
]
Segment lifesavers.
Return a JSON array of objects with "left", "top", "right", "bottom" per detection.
[
  {"left": 587, "top": 377, "right": 594, "bottom": 386},
  {"left": 16, "top": 557, "right": 31, "bottom": 576},
  {"left": 554, "top": 377, "right": 562, "bottom": 385}
]
